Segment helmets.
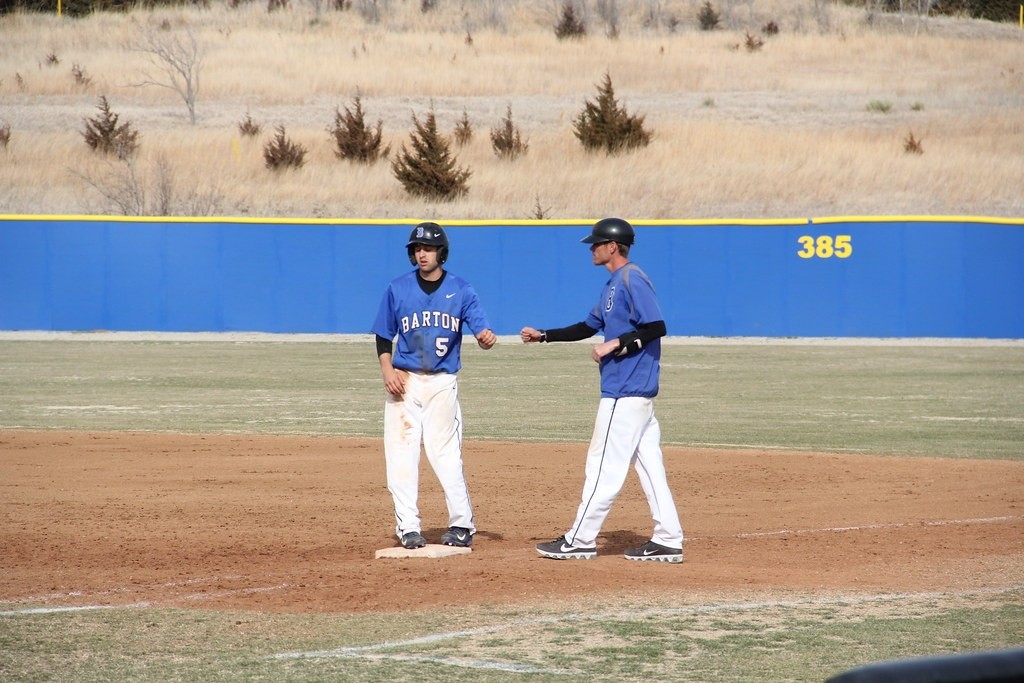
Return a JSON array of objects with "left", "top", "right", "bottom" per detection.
[
  {"left": 582, "top": 219, "right": 634, "bottom": 245},
  {"left": 405, "top": 223, "right": 448, "bottom": 246}
]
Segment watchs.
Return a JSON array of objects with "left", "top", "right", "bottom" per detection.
[{"left": 536, "top": 329, "right": 545, "bottom": 343}]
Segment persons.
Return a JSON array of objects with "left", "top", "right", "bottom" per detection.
[
  {"left": 520, "top": 218, "right": 685, "bottom": 563},
  {"left": 372, "top": 222, "right": 497, "bottom": 548}
]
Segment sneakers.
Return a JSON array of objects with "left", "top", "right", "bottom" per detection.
[
  {"left": 534, "top": 535, "right": 597, "bottom": 559},
  {"left": 442, "top": 526, "right": 473, "bottom": 546},
  {"left": 400, "top": 532, "right": 426, "bottom": 549},
  {"left": 625, "top": 540, "right": 683, "bottom": 564}
]
